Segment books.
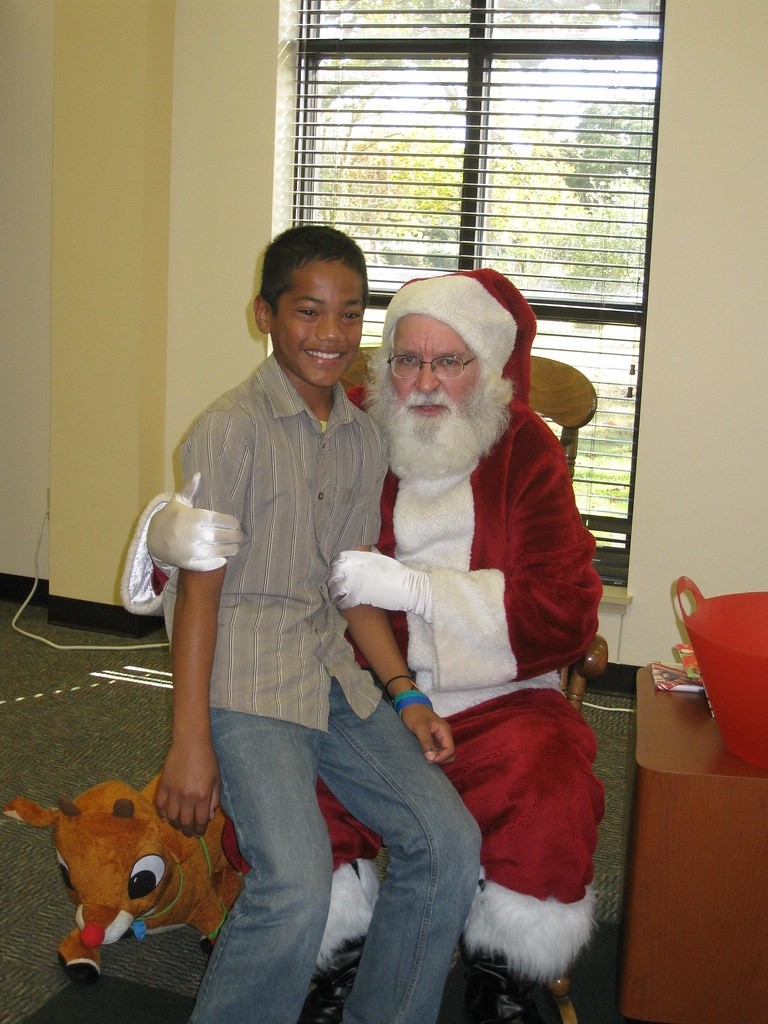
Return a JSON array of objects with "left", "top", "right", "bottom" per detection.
[{"left": 651, "top": 662, "right": 703, "bottom": 692}]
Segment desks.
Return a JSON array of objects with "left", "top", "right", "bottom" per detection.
[{"left": 606, "top": 662, "right": 768, "bottom": 1024}]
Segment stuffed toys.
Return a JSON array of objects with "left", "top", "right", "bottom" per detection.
[{"left": 3, "top": 770, "right": 242, "bottom": 984}]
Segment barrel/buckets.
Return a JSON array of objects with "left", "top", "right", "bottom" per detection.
[{"left": 677, "top": 575, "right": 768, "bottom": 771}]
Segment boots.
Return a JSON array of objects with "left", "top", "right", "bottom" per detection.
[
  {"left": 468, "top": 945, "right": 563, "bottom": 1024},
  {"left": 297, "top": 935, "right": 367, "bottom": 1023}
]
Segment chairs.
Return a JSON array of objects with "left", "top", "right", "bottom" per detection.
[{"left": 336, "top": 345, "right": 612, "bottom": 1024}]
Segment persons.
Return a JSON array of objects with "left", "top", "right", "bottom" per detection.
[
  {"left": 154, "top": 226, "right": 482, "bottom": 1024},
  {"left": 122, "top": 268, "right": 605, "bottom": 1023}
]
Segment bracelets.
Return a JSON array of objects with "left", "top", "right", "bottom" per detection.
[
  {"left": 385, "top": 676, "right": 414, "bottom": 700},
  {"left": 393, "top": 690, "right": 433, "bottom": 718}
]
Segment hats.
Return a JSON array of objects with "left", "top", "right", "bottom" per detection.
[{"left": 382, "top": 267, "right": 537, "bottom": 406}]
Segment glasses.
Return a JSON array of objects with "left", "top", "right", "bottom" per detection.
[{"left": 388, "top": 353, "right": 477, "bottom": 378}]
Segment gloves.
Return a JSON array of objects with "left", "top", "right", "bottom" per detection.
[
  {"left": 147, "top": 472, "right": 243, "bottom": 572},
  {"left": 327, "top": 544, "right": 434, "bottom": 624}
]
